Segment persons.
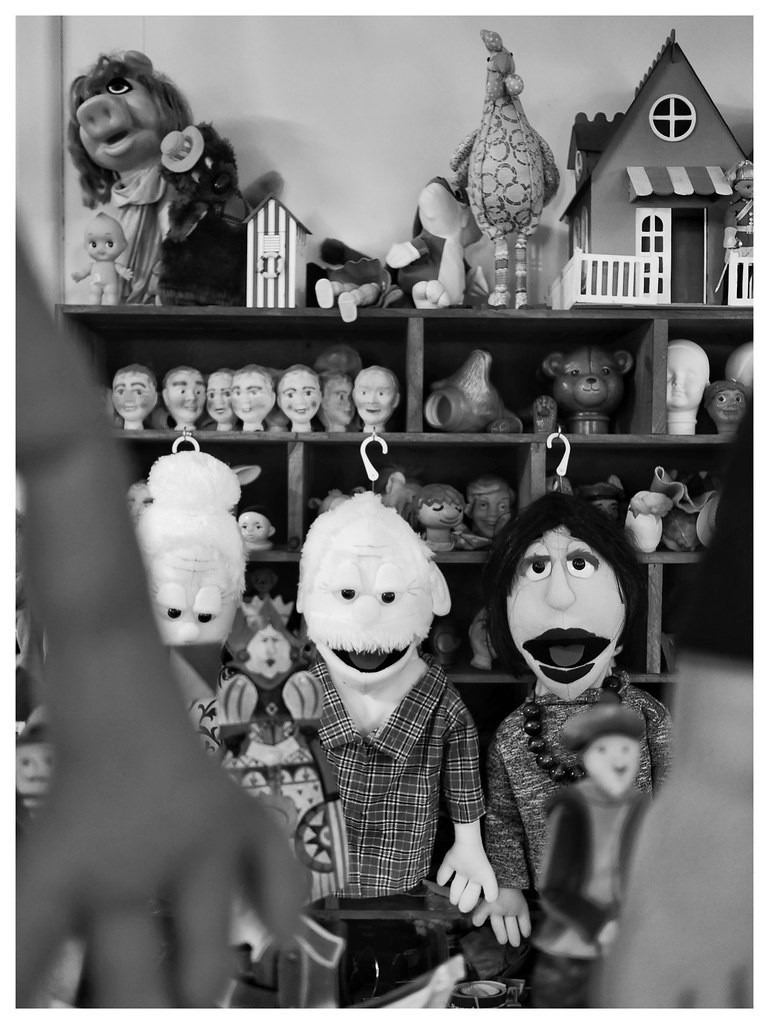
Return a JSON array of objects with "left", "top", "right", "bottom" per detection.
[
  {"left": 135, "top": 451, "right": 248, "bottom": 651},
  {"left": 17, "top": 214, "right": 308, "bottom": 1008},
  {"left": 598, "top": 395, "right": 752, "bottom": 1008},
  {"left": 111, "top": 356, "right": 400, "bottom": 433},
  {"left": 127, "top": 480, "right": 277, "bottom": 548},
  {"left": 484, "top": 492, "right": 675, "bottom": 1007},
  {"left": 723, "top": 158, "right": 753, "bottom": 303},
  {"left": 296, "top": 492, "right": 498, "bottom": 1008},
  {"left": 315, "top": 258, "right": 402, "bottom": 323},
  {"left": 667, "top": 340, "right": 753, "bottom": 435},
  {"left": 310, "top": 468, "right": 514, "bottom": 553},
  {"left": 529, "top": 690, "right": 653, "bottom": 1009},
  {"left": 71, "top": 212, "right": 134, "bottom": 305}
]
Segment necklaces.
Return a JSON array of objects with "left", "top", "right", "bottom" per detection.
[{"left": 524, "top": 674, "right": 621, "bottom": 783}]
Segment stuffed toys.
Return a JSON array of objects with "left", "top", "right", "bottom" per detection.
[
  {"left": 386, "top": 177, "right": 484, "bottom": 310},
  {"left": 159, "top": 122, "right": 257, "bottom": 309}
]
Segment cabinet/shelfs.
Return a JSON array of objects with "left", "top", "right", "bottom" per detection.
[{"left": 55, "top": 304, "right": 753, "bottom": 682}]
